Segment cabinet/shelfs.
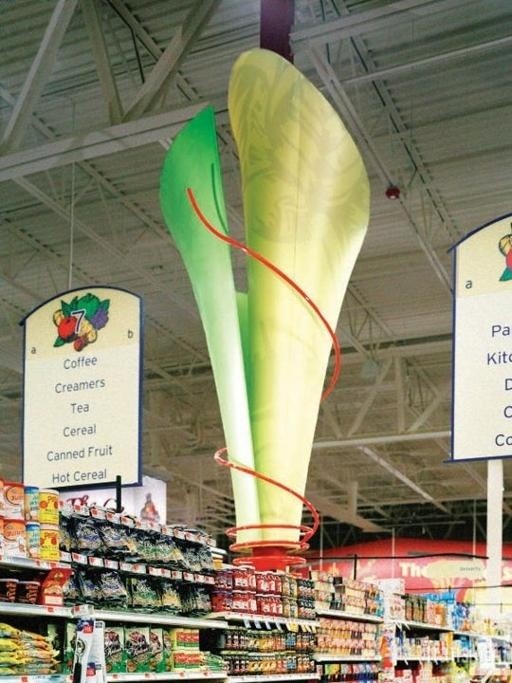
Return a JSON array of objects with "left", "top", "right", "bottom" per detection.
[
  {"left": 453, "top": 631, "right": 509, "bottom": 683},
  {"left": 0, "top": 475, "right": 72, "bottom": 682},
  {"left": 71, "top": 501, "right": 232, "bottom": 682},
  {"left": 314, "top": 605, "right": 384, "bottom": 683},
  {"left": 386, "top": 619, "right": 455, "bottom": 680},
  {"left": 208, "top": 575, "right": 322, "bottom": 681}
]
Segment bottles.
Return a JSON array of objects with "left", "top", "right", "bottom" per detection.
[
  {"left": 24, "top": 485, "right": 40, "bottom": 522},
  {"left": 26, "top": 524, "right": 39, "bottom": 558},
  {"left": 38, "top": 489, "right": 60, "bottom": 524},
  {"left": 4, "top": 520, "right": 25, "bottom": 559},
  {"left": 0, "top": 477, "right": 4, "bottom": 517},
  {"left": 217, "top": 628, "right": 316, "bottom": 674},
  {"left": 0, "top": 516, "right": 4, "bottom": 561},
  {"left": 4, "top": 482, "right": 25, "bottom": 517},
  {"left": 209, "top": 566, "right": 317, "bottom": 620},
  {"left": 40, "top": 524, "right": 61, "bottom": 563}
]
